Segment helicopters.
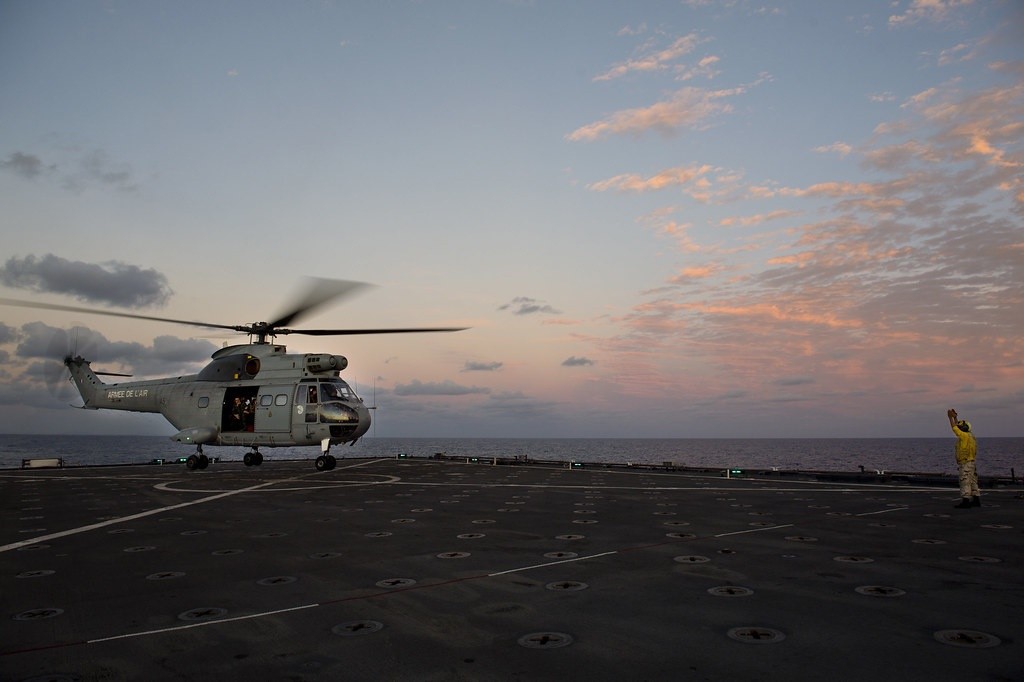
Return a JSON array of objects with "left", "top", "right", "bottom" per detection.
[{"left": 1, "top": 275, "right": 472, "bottom": 472}]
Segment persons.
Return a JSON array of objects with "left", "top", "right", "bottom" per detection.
[
  {"left": 947, "top": 408, "right": 981, "bottom": 508},
  {"left": 310, "top": 388, "right": 344, "bottom": 403},
  {"left": 229, "top": 395, "right": 255, "bottom": 432}
]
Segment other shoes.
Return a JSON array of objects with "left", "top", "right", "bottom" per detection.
[
  {"left": 954, "top": 502, "right": 971, "bottom": 508},
  {"left": 972, "top": 501, "right": 980, "bottom": 506}
]
